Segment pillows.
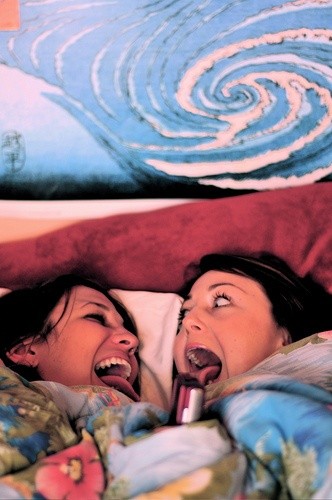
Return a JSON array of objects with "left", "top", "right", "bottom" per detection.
[{"left": 107, "top": 288, "right": 185, "bottom": 413}]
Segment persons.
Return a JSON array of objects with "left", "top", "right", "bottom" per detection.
[
  {"left": 0, "top": 266, "right": 143, "bottom": 403},
  {"left": 172, "top": 251, "right": 332, "bottom": 388}
]
